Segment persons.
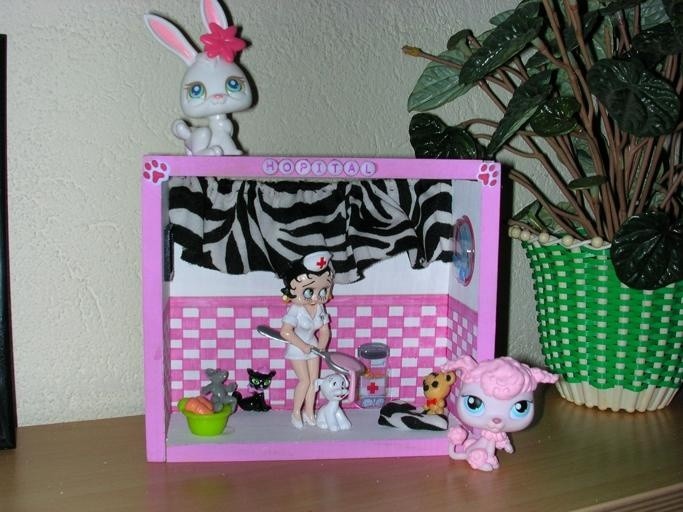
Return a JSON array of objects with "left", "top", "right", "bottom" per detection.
[{"left": 281, "top": 252, "right": 336, "bottom": 427}]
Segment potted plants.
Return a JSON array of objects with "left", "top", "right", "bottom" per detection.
[{"left": 399, "top": 0, "right": 683, "bottom": 415}]
